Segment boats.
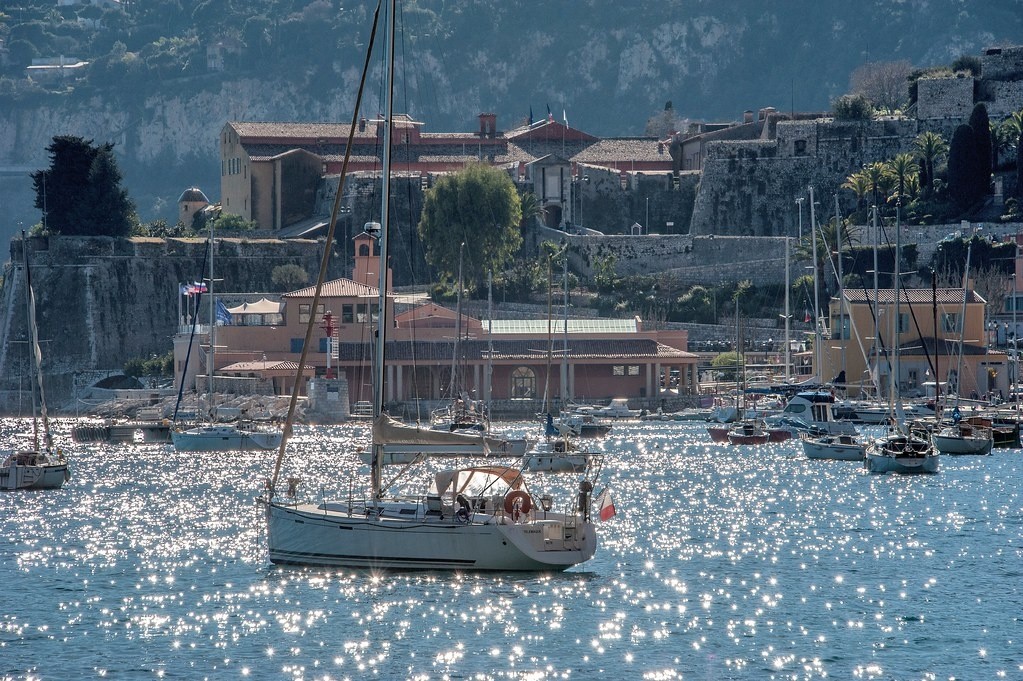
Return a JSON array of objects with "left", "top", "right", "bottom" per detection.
[
  {"left": 799, "top": 432, "right": 866, "bottom": 461},
  {"left": 560, "top": 398, "right": 641, "bottom": 418},
  {"left": 726, "top": 424, "right": 770, "bottom": 445}
]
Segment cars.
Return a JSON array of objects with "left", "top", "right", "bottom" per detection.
[{"left": 746, "top": 376, "right": 768, "bottom": 383}]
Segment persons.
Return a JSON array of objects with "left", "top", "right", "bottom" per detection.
[
  {"left": 983, "top": 390, "right": 992, "bottom": 401},
  {"left": 969, "top": 389, "right": 978, "bottom": 400}
]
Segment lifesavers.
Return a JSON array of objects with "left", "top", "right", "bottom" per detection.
[{"left": 504, "top": 490, "right": 531, "bottom": 513}]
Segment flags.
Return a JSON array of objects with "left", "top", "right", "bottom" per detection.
[
  {"left": 804, "top": 308, "right": 811, "bottom": 323},
  {"left": 547, "top": 104, "right": 555, "bottom": 124},
  {"left": 530, "top": 105, "right": 533, "bottom": 126},
  {"left": 215, "top": 298, "right": 232, "bottom": 324},
  {"left": 562, "top": 105, "right": 570, "bottom": 130},
  {"left": 595, "top": 487, "right": 617, "bottom": 522},
  {"left": 180, "top": 281, "right": 208, "bottom": 298}
]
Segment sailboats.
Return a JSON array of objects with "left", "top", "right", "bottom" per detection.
[
  {"left": 521, "top": 256, "right": 602, "bottom": 475},
  {"left": 539, "top": 260, "right": 613, "bottom": 439},
  {"left": 170, "top": 220, "right": 283, "bottom": 453},
  {"left": 359, "top": 270, "right": 536, "bottom": 463},
  {"left": 706, "top": 298, "right": 793, "bottom": 445},
  {"left": 0, "top": 229, "right": 71, "bottom": 491},
  {"left": 754, "top": 183, "right": 1023, "bottom": 457},
  {"left": 864, "top": 198, "right": 940, "bottom": 476},
  {"left": 430, "top": 243, "right": 489, "bottom": 433},
  {"left": 253, "top": 1, "right": 617, "bottom": 574}
]
